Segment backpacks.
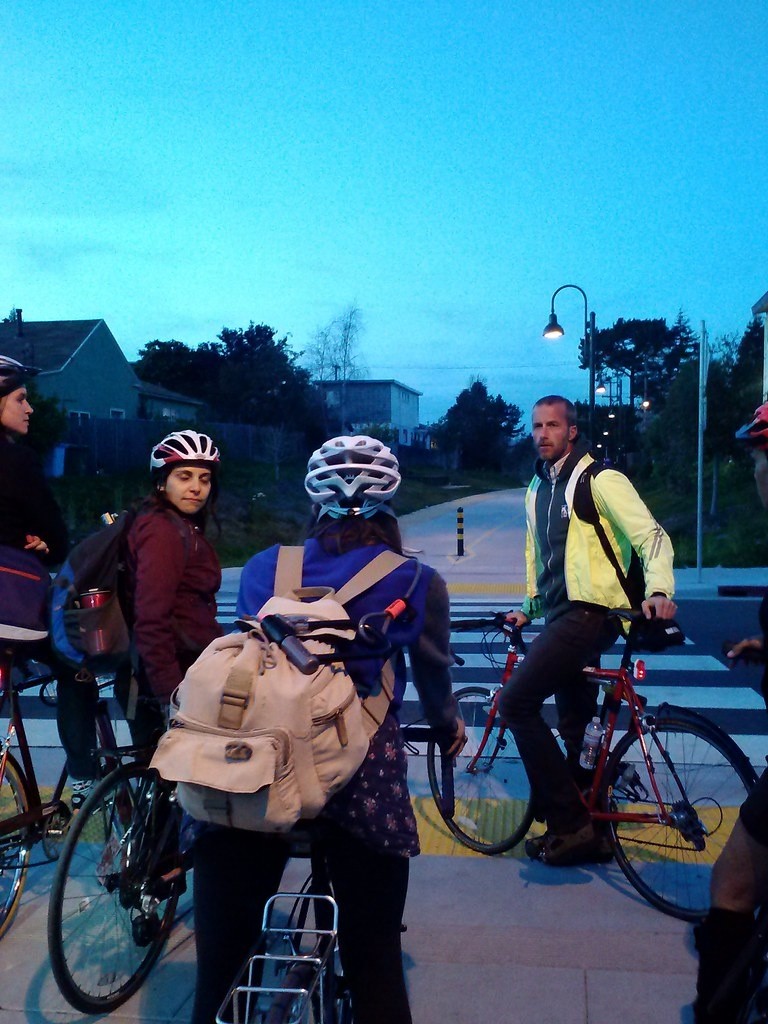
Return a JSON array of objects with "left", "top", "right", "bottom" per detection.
[
  {"left": 46, "top": 499, "right": 190, "bottom": 698},
  {"left": 146, "top": 546, "right": 407, "bottom": 835},
  {"left": 579, "top": 460, "right": 675, "bottom": 650}
]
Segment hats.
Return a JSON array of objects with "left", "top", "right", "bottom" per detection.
[{"left": 303, "top": 435, "right": 402, "bottom": 521}]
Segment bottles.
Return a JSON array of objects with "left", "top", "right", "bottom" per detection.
[{"left": 579, "top": 716, "right": 603, "bottom": 770}]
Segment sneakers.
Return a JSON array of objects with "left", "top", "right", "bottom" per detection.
[
  {"left": 522, "top": 816, "right": 615, "bottom": 865},
  {"left": 71, "top": 769, "right": 126, "bottom": 815}
]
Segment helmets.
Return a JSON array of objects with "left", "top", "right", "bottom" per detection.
[
  {"left": 150, "top": 430, "right": 223, "bottom": 482},
  {"left": 0, "top": 354, "right": 43, "bottom": 397},
  {"left": 736, "top": 402, "right": 768, "bottom": 454}
]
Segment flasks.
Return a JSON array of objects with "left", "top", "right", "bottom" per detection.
[{"left": 79, "top": 587, "right": 112, "bottom": 656}]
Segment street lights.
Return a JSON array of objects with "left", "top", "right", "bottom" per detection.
[
  {"left": 642, "top": 401, "right": 649, "bottom": 427},
  {"left": 596, "top": 378, "right": 623, "bottom": 472},
  {"left": 542, "top": 284, "right": 596, "bottom": 452}
]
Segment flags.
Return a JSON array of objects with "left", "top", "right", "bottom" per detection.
[{"left": 703, "top": 330, "right": 711, "bottom": 422}]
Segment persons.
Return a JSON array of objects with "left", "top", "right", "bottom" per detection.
[
  {"left": 193, "top": 435, "right": 467, "bottom": 1024},
  {"left": 1, "top": 354, "right": 123, "bottom": 814},
  {"left": 693, "top": 401, "right": 768, "bottom": 1024},
  {"left": 496, "top": 395, "right": 677, "bottom": 863},
  {"left": 126, "top": 429, "right": 221, "bottom": 944}
]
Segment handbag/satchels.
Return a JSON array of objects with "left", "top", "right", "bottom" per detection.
[
  {"left": 0, "top": 547, "right": 53, "bottom": 645},
  {"left": 687, "top": 905, "right": 768, "bottom": 1024}
]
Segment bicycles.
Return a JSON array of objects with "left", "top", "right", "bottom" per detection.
[
  {"left": 426, "top": 605, "right": 763, "bottom": 923},
  {"left": 0, "top": 659, "right": 196, "bottom": 1017},
  {"left": 213, "top": 720, "right": 459, "bottom": 1024}
]
